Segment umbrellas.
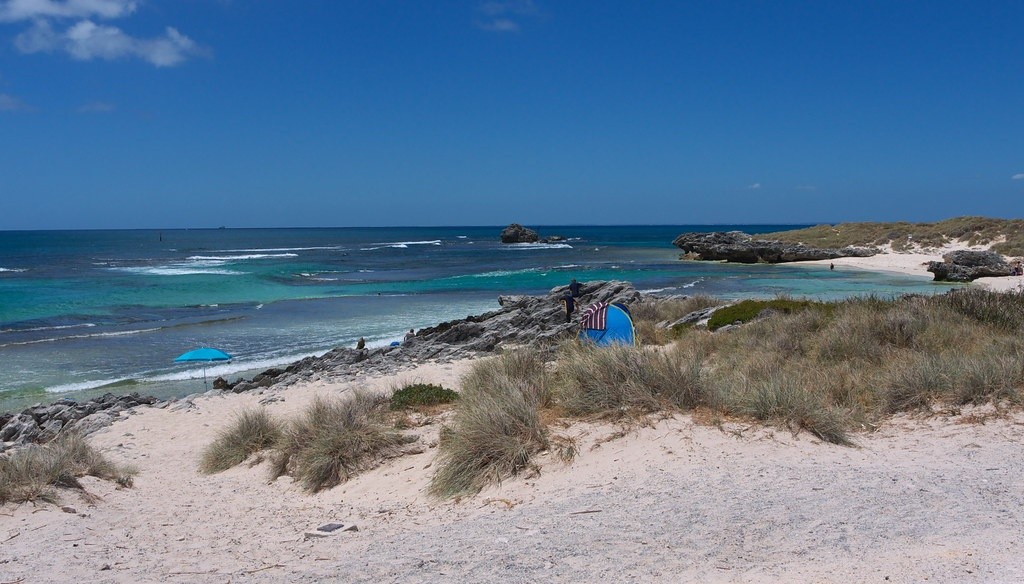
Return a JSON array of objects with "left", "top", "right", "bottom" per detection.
[{"left": 173, "top": 346, "right": 232, "bottom": 392}]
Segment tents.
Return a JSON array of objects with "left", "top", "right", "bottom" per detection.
[{"left": 574, "top": 303, "right": 636, "bottom": 349}]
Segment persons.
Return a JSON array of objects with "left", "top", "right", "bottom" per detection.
[
  {"left": 356, "top": 337, "right": 365, "bottom": 349},
  {"left": 566, "top": 279, "right": 583, "bottom": 311},
  {"left": 1015, "top": 261, "right": 1021, "bottom": 276},
  {"left": 830, "top": 263, "right": 834, "bottom": 270},
  {"left": 559, "top": 291, "right": 578, "bottom": 323},
  {"left": 404, "top": 329, "right": 415, "bottom": 342}
]
